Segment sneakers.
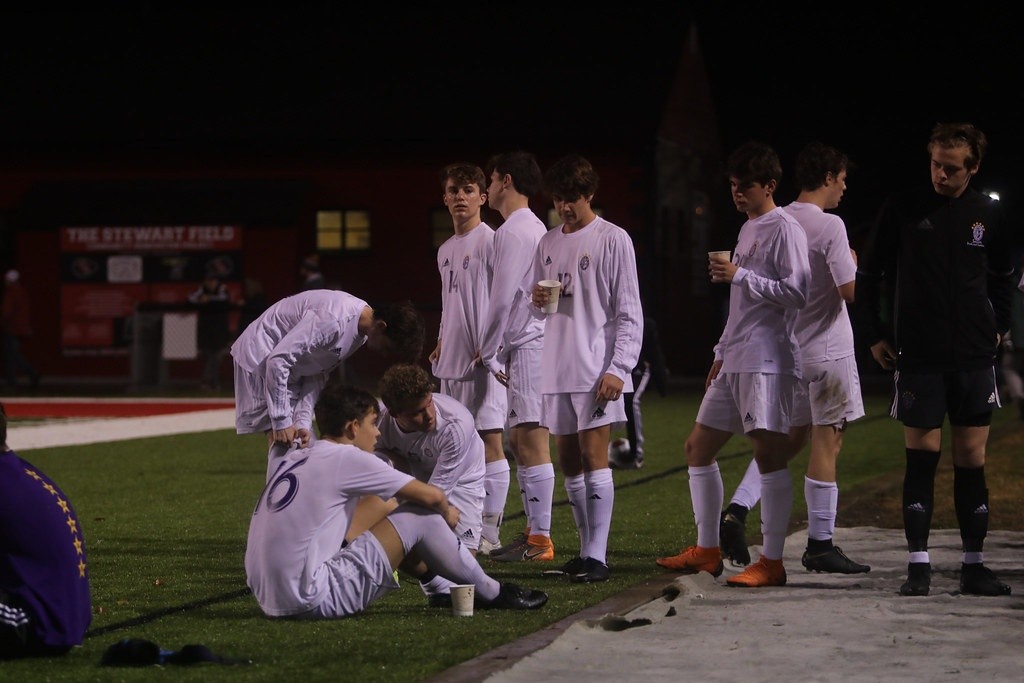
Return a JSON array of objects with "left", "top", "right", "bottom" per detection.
[
  {"left": 489, "top": 532, "right": 528, "bottom": 557},
  {"left": 571, "top": 556, "right": 610, "bottom": 584},
  {"left": 490, "top": 542, "right": 554, "bottom": 562},
  {"left": 727, "top": 556, "right": 786, "bottom": 587},
  {"left": 801, "top": 546, "right": 870, "bottom": 574},
  {"left": 656, "top": 545, "right": 723, "bottom": 577},
  {"left": 477, "top": 534, "right": 503, "bottom": 556},
  {"left": 542, "top": 555, "right": 586, "bottom": 578},
  {"left": 718, "top": 503, "right": 751, "bottom": 566},
  {"left": 960, "top": 561, "right": 1011, "bottom": 595},
  {"left": 428, "top": 592, "right": 453, "bottom": 611},
  {"left": 900, "top": 561, "right": 931, "bottom": 596},
  {"left": 476, "top": 580, "right": 548, "bottom": 611}
]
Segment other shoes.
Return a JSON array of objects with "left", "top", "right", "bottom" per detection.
[{"left": 619, "top": 457, "right": 644, "bottom": 469}]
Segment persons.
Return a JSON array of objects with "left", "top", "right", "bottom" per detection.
[
  {"left": 428, "top": 163, "right": 511, "bottom": 557},
  {"left": 720, "top": 147, "right": 870, "bottom": 575},
  {"left": 655, "top": 146, "right": 811, "bottom": 585},
  {"left": 245, "top": 386, "right": 549, "bottom": 619},
  {"left": 618, "top": 278, "right": 652, "bottom": 467},
  {"left": 851, "top": 124, "right": 1024, "bottom": 595},
  {"left": 189, "top": 271, "right": 229, "bottom": 396},
  {"left": 368, "top": 363, "right": 487, "bottom": 560},
  {"left": 477, "top": 153, "right": 554, "bottom": 565},
  {"left": 302, "top": 258, "right": 324, "bottom": 290},
  {"left": 1004, "top": 257, "right": 1024, "bottom": 418},
  {"left": 0, "top": 269, "right": 39, "bottom": 397},
  {"left": 229, "top": 289, "right": 425, "bottom": 490},
  {"left": 0, "top": 402, "right": 93, "bottom": 659},
  {"left": 533, "top": 155, "right": 645, "bottom": 584}
]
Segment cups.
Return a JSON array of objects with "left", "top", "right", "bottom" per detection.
[
  {"left": 538, "top": 280, "right": 561, "bottom": 313},
  {"left": 709, "top": 251, "right": 730, "bottom": 279},
  {"left": 449, "top": 585, "right": 474, "bottom": 615}
]
